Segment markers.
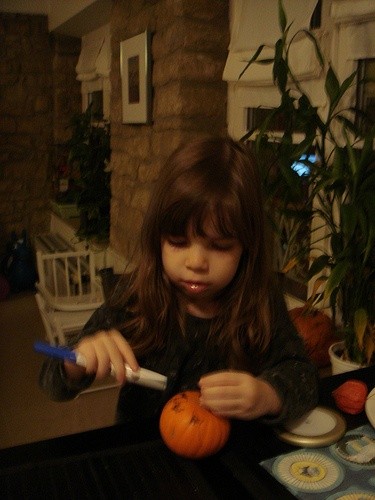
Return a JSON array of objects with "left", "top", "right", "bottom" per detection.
[{"left": 32, "top": 343, "right": 179, "bottom": 393}]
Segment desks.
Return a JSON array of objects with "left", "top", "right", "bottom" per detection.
[{"left": 0, "top": 364, "right": 375, "bottom": 500}]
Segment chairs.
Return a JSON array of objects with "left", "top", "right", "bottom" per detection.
[{"left": 33, "top": 250, "right": 123, "bottom": 397}]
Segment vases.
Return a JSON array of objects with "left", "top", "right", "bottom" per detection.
[{"left": 49, "top": 198, "right": 78, "bottom": 218}]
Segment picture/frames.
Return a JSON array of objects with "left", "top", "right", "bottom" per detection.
[{"left": 119, "top": 30, "right": 152, "bottom": 127}]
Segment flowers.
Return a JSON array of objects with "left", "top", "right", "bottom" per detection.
[{"left": 48, "top": 163, "right": 80, "bottom": 204}]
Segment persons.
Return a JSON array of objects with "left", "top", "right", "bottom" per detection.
[{"left": 38, "top": 137, "right": 318, "bottom": 424}]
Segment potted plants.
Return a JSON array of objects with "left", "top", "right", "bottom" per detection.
[
  {"left": 237, "top": 0, "right": 375, "bottom": 377},
  {"left": 68, "top": 101, "right": 111, "bottom": 251}
]
